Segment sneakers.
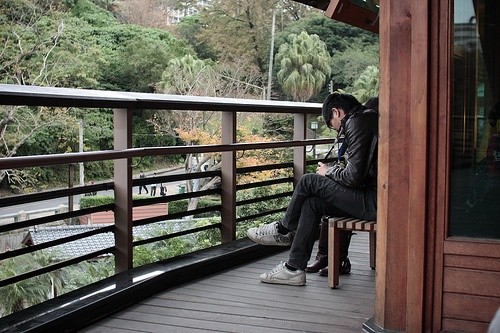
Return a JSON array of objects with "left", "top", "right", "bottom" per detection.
[
  {"left": 247, "top": 221, "right": 294, "bottom": 246},
  {"left": 260, "top": 261, "right": 306, "bottom": 285}
]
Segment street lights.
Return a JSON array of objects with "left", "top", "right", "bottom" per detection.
[
  {"left": 265, "top": 4, "right": 294, "bottom": 100},
  {"left": 310, "top": 121, "right": 319, "bottom": 157}
]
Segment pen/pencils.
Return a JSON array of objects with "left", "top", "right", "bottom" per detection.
[{"left": 332, "top": 161, "right": 336, "bottom": 166}]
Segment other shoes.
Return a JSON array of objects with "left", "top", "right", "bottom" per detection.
[
  {"left": 318, "top": 258, "right": 351, "bottom": 275},
  {"left": 305, "top": 254, "right": 328, "bottom": 272}
]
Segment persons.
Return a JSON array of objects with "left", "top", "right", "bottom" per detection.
[
  {"left": 138, "top": 171, "right": 148, "bottom": 194},
  {"left": 149, "top": 173, "right": 159, "bottom": 196},
  {"left": 247, "top": 93, "right": 377, "bottom": 286},
  {"left": 305, "top": 97, "right": 377, "bottom": 276},
  {"left": 84, "top": 180, "right": 97, "bottom": 196}
]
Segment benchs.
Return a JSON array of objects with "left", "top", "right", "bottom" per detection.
[{"left": 328, "top": 217, "right": 377, "bottom": 290}]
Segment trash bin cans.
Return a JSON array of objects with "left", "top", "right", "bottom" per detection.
[{"left": 179, "top": 185, "right": 185, "bottom": 193}]
